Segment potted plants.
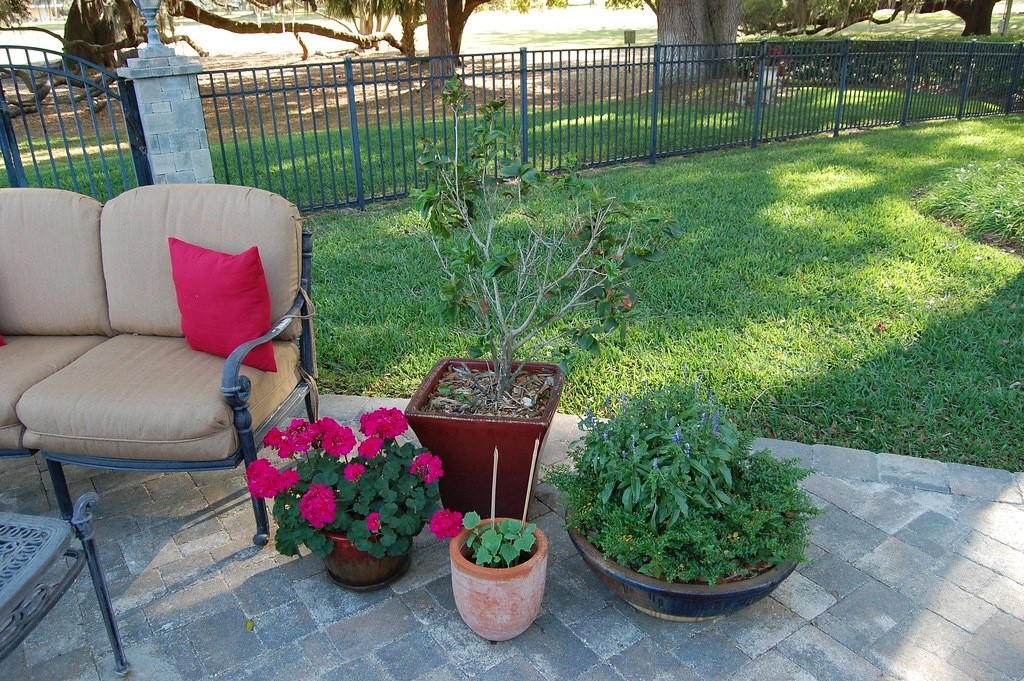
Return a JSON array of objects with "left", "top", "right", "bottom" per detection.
[
  {"left": 383, "top": 73, "right": 682, "bottom": 516},
  {"left": 450, "top": 512, "right": 548, "bottom": 643}
]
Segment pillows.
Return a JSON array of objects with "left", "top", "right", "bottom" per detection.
[{"left": 168, "top": 237, "right": 280, "bottom": 373}]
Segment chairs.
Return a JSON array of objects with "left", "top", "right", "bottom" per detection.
[{"left": 0, "top": 184, "right": 321, "bottom": 681}]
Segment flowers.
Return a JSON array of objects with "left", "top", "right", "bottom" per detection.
[
  {"left": 545, "top": 364, "right": 824, "bottom": 583},
  {"left": 247, "top": 408, "right": 465, "bottom": 562}
]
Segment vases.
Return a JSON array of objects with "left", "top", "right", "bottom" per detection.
[
  {"left": 320, "top": 530, "right": 413, "bottom": 592},
  {"left": 565, "top": 503, "right": 806, "bottom": 622}
]
